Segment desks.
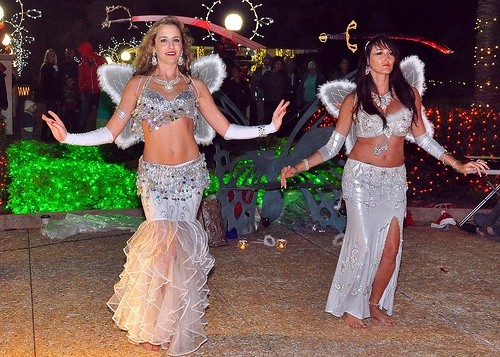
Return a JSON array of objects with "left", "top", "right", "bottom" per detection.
[{"left": 459, "top": 169, "right": 500, "bottom": 228}]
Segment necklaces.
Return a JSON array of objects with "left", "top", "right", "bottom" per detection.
[
  {"left": 153, "top": 76, "right": 180, "bottom": 92},
  {"left": 372, "top": 88, "right": 395, "bottom": 112}
]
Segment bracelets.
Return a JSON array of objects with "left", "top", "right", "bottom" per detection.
[
  {"left": 451, "top": 160, "right": 459, "bottom": 169},
  {"left": 442, "top": 152, "right": 452, "bottom": 165},
  {"left": 258, "top": 125, "right": 267, "bottom": 137},
  {"left": 301, "top": 159, "right": 310, "bottom": 171}
]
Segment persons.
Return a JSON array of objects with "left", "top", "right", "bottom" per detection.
[
  {"left": 39, "top": 42, "right": 107, "bottom": 146},
  {"left": 42, "top": 16, "right": 290, "bottom": 357},
  {"left": 281, "top": 37, "right": 491, "bottom": 328},
  {"left": 220, "top": 53, "right": 349, "bottom": 151}
]
